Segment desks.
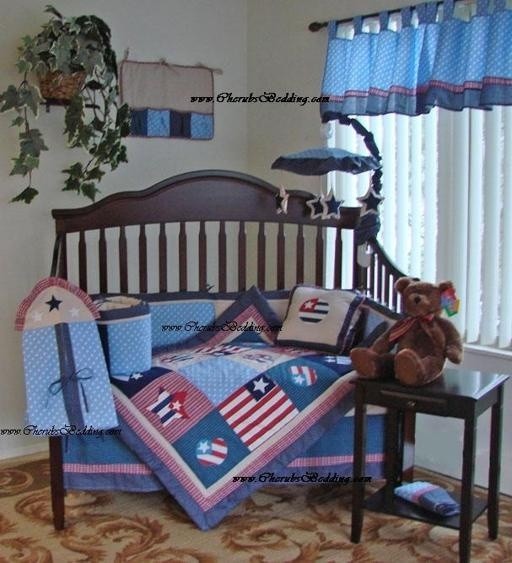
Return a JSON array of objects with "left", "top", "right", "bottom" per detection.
[{"left": 351, "top": 371, "right": 510, "bottom": 563}]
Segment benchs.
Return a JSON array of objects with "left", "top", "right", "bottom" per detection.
[{"left": 48, "top": 167, "right": 423, "bottom": 529}]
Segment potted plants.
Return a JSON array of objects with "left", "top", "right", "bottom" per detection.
[{"left": 1, "top": 4, "right": 132, "bottom": 203}]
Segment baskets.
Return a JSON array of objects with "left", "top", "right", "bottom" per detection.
[{"left": 37, "top": 63, "right": 86, "bottom": 101}]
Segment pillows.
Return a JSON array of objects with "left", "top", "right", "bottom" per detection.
[{"left": 276, "top": 284, "right": 367, "bottom": 356}]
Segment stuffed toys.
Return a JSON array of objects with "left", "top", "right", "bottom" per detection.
[{"left": 349, "top": 277, "right": 462, "bottom": 388}]
